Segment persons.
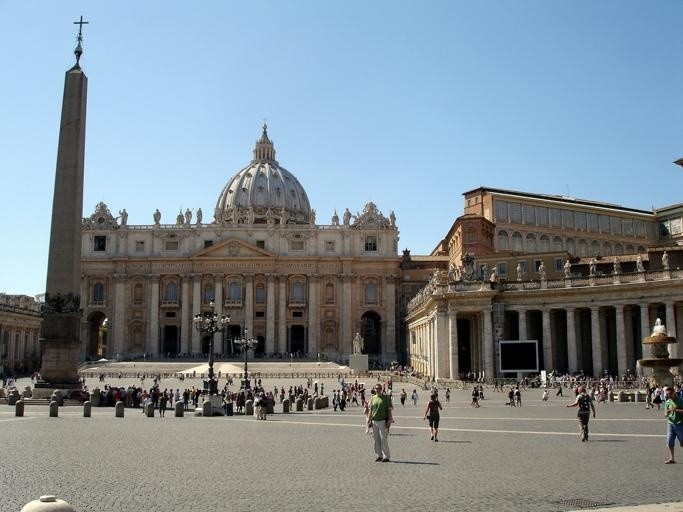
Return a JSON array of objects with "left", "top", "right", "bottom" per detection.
[
  {"left": 563, "top": 260, "right": 571, "bottom": 278},
  {"left": 352, "top": 332, "right": 364, "bottom": 356},
  {"left": 196, "top": 208, "right": 202, "bottom": 224},
  {"left": 118, "top": 208, "right": 128, "bottom": 225},
  {"left": 472, "top": 370, "right": 682, "bottom": 411},
  {"left": 152, "top": 209, "right": 160, "bottom": 225},
  {"left": 184, "top": 208, "right": 194, "bottom": 224},
  {"left": 424, "top": 394, "right": 443, "bottom": 442},
  {"left": 664, "top": 387, "right": 683, "bottom": 464},
  {"left": 390, "top": 211, "right": 396, "bottom": 226},
  {"left": 482, "top": 263, "right": 489, "bottom": 279},
  {"left": 368, "top": 384, "right": 393, "bottom": 462},
  {"left": 430, "top": 386, "right": 451, "bottom": 403},
  {"left": 636, "top": 253, "right": 646, "bottom": 272},
  {"left": 539, "top": 262, "right": 546, "bottom": 279},
  {"left": 343, "top": 208, "right": 351, "bottom": 225},
  {"left": 2, "top": 353, "right": 418, "bottom": 421},
  {"left": 589, "top": 259, "right": 596, "bottom": 276},
  {"left": 516, "top": 263, "right": 522, "bottom": 281},
  {"left": 614, "top": 257, "right": 622, "bottom": 274},
  {"left": 662, "top": 251, "right": 670, "bottom": 270},
  {"left": 566, "top": 386, "right": 595, "bottom": 441},
  {"left": 309, "top": 209, "right": 316, "bottom": 224}
]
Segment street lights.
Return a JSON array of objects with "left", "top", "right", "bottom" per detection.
[
  {"left": 234, "top": 328, "right": 258, "bottom": 390},
  {"left": 193, "top": 298, "right": 230, "bottom": 394}
]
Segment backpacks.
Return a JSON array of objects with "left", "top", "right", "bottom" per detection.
[{"left": 578, "top": 393, "right": 589, "bottom": 410}]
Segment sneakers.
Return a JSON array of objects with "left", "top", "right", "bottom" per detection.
[
  {"left": 374, "top": 457, "right": 389, "bottom": 462},
  {"left": 430, "top": 436, "right": 439, "bottom": 442},
  {"left": 664, "top": 459, "right": 677, "bottom": 464}
]
